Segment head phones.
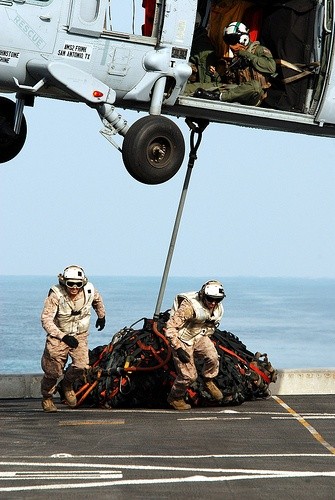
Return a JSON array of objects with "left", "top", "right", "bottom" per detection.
[
  {"left": 198, "top": 281, "right": 218, "bottom": 300},
  {"left": 59, "top": 275, "right": 87, "bottom": 288},
  {"left": 224, "top": 26, "right": 250, "bottom": 45}
]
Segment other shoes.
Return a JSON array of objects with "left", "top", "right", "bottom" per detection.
[
  {"left": 58, "top": 380, "right": 77, "bottom": 406},
  {"left": 41, "top": 397, "right": 57, "bottom": 412},
  {"left": 167, "top": 393, "right": 191, "bottom": 410},
  {"left": 195, "top": 88, "right": 220, "bottom": 101},
  {"left": 203, "top": 379, "right": 223, "bottom": 400}
]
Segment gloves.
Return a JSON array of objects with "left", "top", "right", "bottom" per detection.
[
  {"left": 176, "top": 348, "right": 190, "bottom": 363},
  {"left": 62, "top": 335, "right": 79, "bottom": 348},
  {"left": 95, "top": 316, "right": 105, "bottom": 331}
]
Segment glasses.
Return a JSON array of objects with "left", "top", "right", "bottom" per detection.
[
  {"left": 204, "top": 296, "right": 224, "bottom": 303},
  {"left": 63, "top": 280, "right": 84, "bottom": 289}
]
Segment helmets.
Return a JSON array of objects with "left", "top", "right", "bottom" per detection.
[
  {"left": 224, "top": 22, "right": 250, "bottom": 45},
  {"left": 202, "top": 280, "right": 225, "bottom": 298},
  {"left": 62, "top": 265, "right": 84, "bottom": 280}
]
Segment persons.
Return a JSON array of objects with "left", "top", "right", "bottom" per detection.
[
  {"left": 184, "top": 22, "right": 276, "bottom": 105},
  {"left": 165, "top": 280, "right": 226, "bottom": 411},
  {"left": 41, "top": 265, "right": 106, "bottom": 411},
  {"left": 189, "top": 23, "right": 221, "bottom": 83}
]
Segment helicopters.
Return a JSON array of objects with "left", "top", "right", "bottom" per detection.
[{"left": 0, "top": 0, "right": 335, "bottom": 186}]
